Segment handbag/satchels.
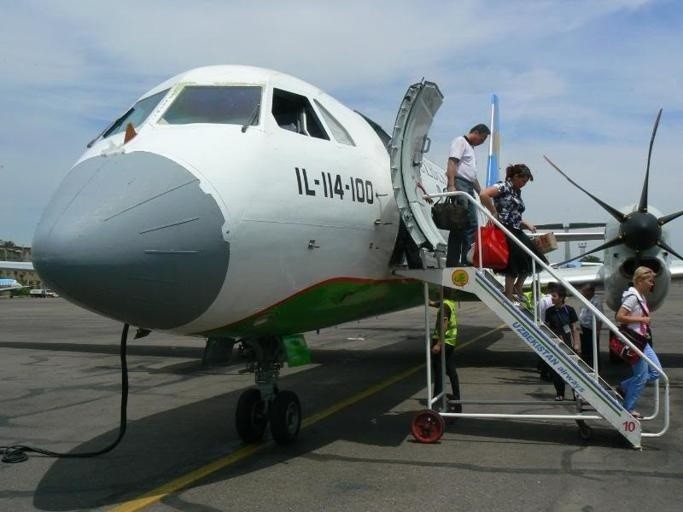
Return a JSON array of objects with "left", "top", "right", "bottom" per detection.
[
  {"left": 607, "top": 323, "right": 651, "bottom": 364},
  {"left": 432, "top": 192, "right": 472, "bottom": 232},
  {"left": 473, "top": 218, "right": 512, "bottom": 271}
]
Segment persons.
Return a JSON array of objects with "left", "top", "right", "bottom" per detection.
[
  {"left": 446, "top": 123, "right": 492, "bottom": 268},
  {"left": 615, "top": 266, "right": 663, "bottom": 418},
  {"left": 429, "top": 284, "right": 462, "bottom": 412},
  {"left": 480, "top": 164, "right": 549, "bottom": 307},
  {"left": 537, "top": 282, "right": 557, "bottom": 381},
  {"left": 523, "top": 280, "right": 545, "bottom": 309},
  {"left": 578, "top": 282, "right": 604, "bottom": 374},
  {"left": 545, "top": 285, "right": 582, "bottom": 401}
]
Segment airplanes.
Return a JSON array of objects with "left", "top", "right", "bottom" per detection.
[
  {"left": 0, "top": 278, "right": 29, "bottom": 298},
  {"left": 31, "top": 64, "right": 682, "bottom": 450},
  {"left": 494, "top": 109, "right": 682, "bottom": 314}
]
